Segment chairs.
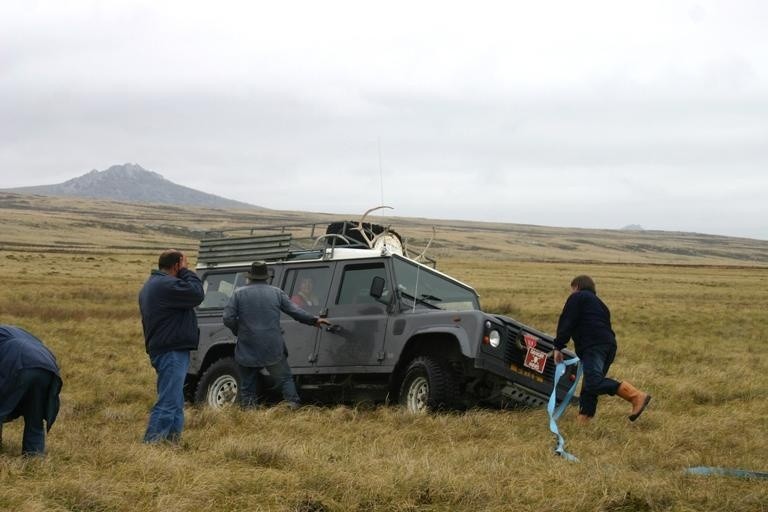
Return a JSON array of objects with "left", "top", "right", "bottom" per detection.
[{"left": 197, "top": 290, "right": 233, "bottom": 310}]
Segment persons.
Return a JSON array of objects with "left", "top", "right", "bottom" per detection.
[
  {"left": 0, "top": 326, "right": 63, "bottom": 459},
  {"left": 291, "top": 275, "right": 321, "bottom": 308},
  {"left": 223, "top": 261, "right": 332, "bottom": 412},
  {"left": 554, "top": 276, "right": 652, "bottom": 421},
  {"left": 139, "top": 249, "right": 204, "bottom": 450}
]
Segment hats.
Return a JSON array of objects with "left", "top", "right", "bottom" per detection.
[{"left": 243, "top": 261, "right": 273, "bottom": 280}]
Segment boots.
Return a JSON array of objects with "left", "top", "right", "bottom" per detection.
[
  {"left": 576, "top": 415, "right": 591, "bottom": 424},
  {"left": 615, "top": 380, "right": 652, "bottom": 422}
]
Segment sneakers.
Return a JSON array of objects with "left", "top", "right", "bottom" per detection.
[{"left": 166, "top": 433, "right": 189, "bottom": 450}]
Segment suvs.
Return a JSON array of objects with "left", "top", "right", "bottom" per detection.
[{"left": 187, "top": 253, "right": 578, "bottom": 420}]
